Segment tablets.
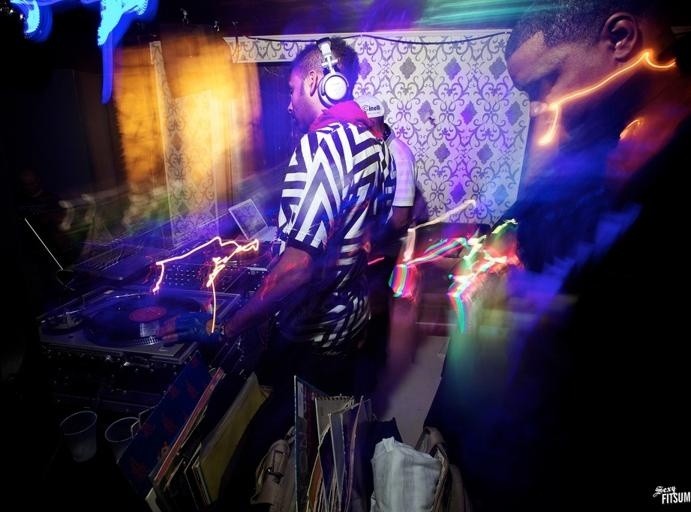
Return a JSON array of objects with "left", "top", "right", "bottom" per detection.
[{"left": 228, "top": 198, "right": 277, "bottom": 241}]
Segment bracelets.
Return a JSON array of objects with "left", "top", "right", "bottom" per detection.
[{"left": 218, "top": 318, "right": 228, "bottom": 339}]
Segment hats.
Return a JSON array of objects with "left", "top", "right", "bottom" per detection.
[{"left": 355, "top": 95, "right": 385, "bottom": 119}]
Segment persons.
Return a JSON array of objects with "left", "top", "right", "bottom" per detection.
[
  {"left": 156, "top": 38, "right": 397, "bottom": 511},
  {"left": 14, "top": 165, "right": 60, "bottom": 264},
  {"left": 354, "top": 93, "right": 415, "bottom": 414},
  {"left": 456, "top": 0, "right": 691, "bottom": 511}
]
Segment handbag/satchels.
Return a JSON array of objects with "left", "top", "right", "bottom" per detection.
[
  {"left": 253, "top": 424, "right": 448, "bottom": 512},
  {"left": 372, "top": 437, "right": 442, "bottom": 512}
]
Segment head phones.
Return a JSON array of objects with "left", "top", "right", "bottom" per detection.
[{"left": 316, "top": 37, "right": 350, "bottom": 107}]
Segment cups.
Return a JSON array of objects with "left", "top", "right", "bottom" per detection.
[
  {"left": 105, "top": 417, "right": 141, "bottom": 464},
  {"left": 57, "top": 410, "right": 97, "bottom": 462}
]
